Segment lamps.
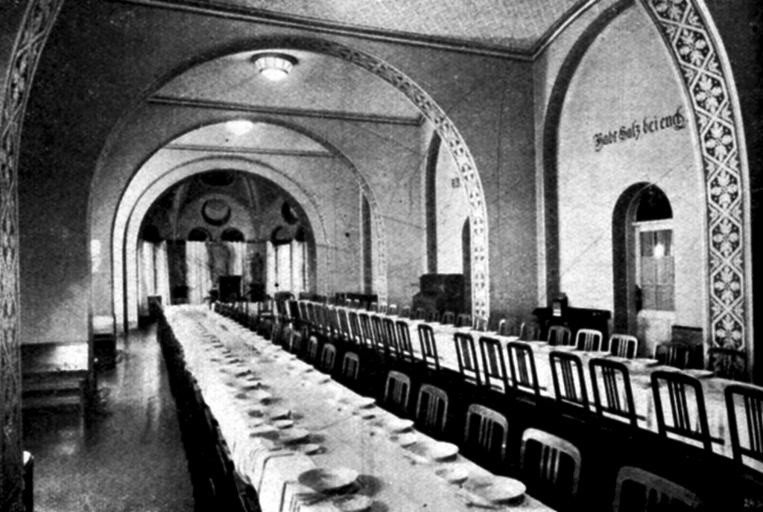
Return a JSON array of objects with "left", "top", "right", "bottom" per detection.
[{"left": 250, "top": 52, "right": 298, "bottom": 83}]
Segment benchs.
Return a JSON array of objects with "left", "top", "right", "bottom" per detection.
[{"left": 22, "top": 343, "right": 91, "bottom": 440}]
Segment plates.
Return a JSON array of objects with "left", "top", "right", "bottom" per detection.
[
  {"left": 297, "top": 466, "right": 358, "bottom": 492},
  {"left": 522, "top": 340, "right": 716, "bottom": 379},
  {"left": 331, "top": 492, "right": 371, "bottom": 512},
  {"left": 203, "top": 361, "right": 417, "bottom": 454},
  {"left": 424, "top": 439, "right": 459, "bottom": 460},
  {"left": 432, "top": 462, "right": 468, "bottom": 484},
  {"left": 461, "top": 472, "right": 528, "bottom": 504}
]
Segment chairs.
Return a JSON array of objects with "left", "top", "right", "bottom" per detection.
[
  {"left": 382, "top": 311, "right": 762, "bottom": 512},
  {"left": 215, "top": 295, "right": 442, "bottom": 392}
]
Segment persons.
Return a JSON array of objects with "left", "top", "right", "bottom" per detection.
[{"left": 201, "top": 283, "right": 218, "bottom": 308}]
